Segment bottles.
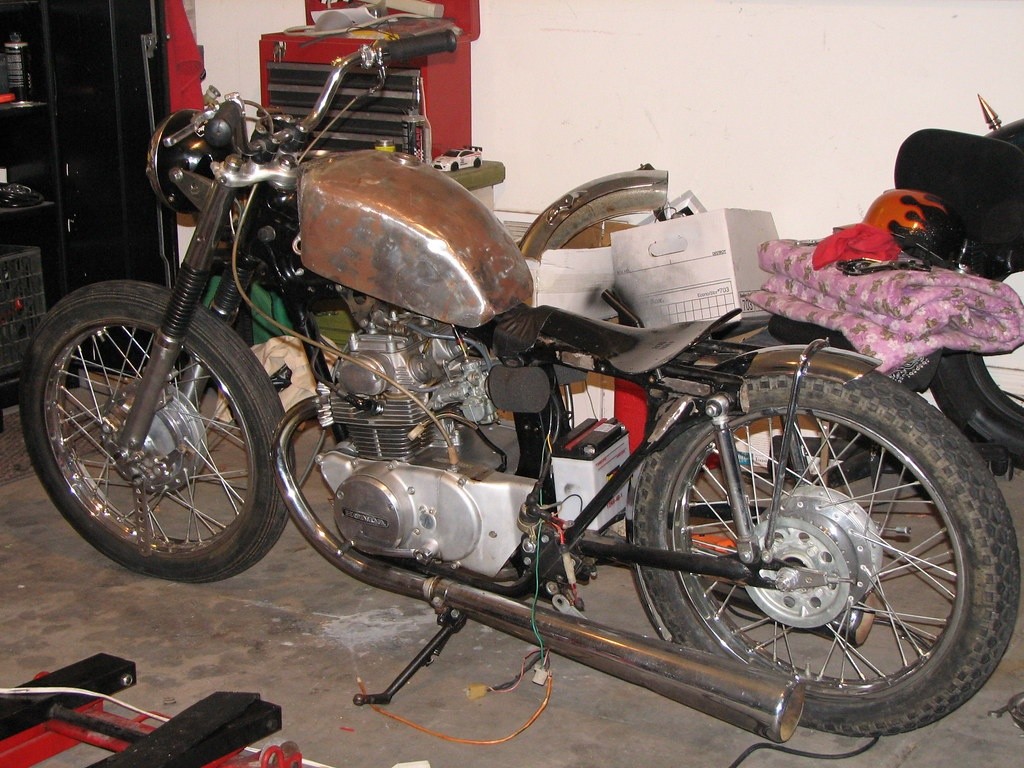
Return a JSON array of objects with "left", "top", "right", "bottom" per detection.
[{"left": 408, "top": 76, "right": 433, "bottom": 168}]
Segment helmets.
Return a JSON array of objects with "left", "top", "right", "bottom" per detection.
[{"left": 862, "top": 189, "right": 959, "bottom": 256}]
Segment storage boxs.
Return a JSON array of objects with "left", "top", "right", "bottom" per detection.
[
  {"left": 607, "top": 206, "right": 779, "bottom": 322},
  {"left": 524, "top": 220, "right": 640, "bottom": 318},
  {"left": 0, "top": 244, "right": 47, "bottom": 376}
]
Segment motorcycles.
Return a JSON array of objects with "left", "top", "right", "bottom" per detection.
[{"left": 15, "top": 23, "right": 1024, "bottom": 749}]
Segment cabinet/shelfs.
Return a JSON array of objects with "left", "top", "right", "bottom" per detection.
[{"left": 0, "top": 0, "right": 81, "bottom": 410}]
